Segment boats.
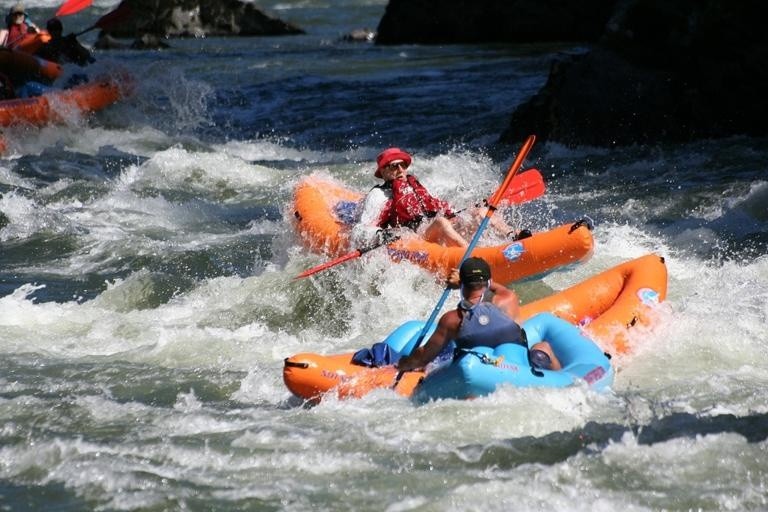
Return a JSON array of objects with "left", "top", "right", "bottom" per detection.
[
  {"left": 0, "top": 63, "right": 140, "bottom": 131},
  {"left": 287, "top": 172, "right": 600, "bottom": 290},
  {"left": 278, "top": 250, "right": 667, "bottom": 403},
  {"left": 381, "top": 305, "right": 620, "bottom": 408}
]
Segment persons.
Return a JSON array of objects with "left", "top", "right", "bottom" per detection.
[
  {"left": 351, "top": 147, "right": 533, "bottom": 250},
  {"left": 0, "top": 69, "right": 62, "bottom": 102},
  {"left": 32, "top": 16, "right": 96, "bottom": 69},
  {"left": 395, "top": 256, "right": 564, "bottom": 374},
  {"left": 0, "top": 3, "right": 42, "bottom": 49}
]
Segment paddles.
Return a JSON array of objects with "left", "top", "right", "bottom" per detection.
[
  {"left": 394, "top": 135, "right": 536, "bottom": 388},
  {"left": 4, "top": 1, "right": 93, "bottom": 47},
  {"left": 42, "top": 7, "right": 125, "bottom": 45},
  {"left": 293, "top": 169, "right": 545, "bottom": 280}
]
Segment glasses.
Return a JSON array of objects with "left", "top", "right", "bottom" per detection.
[{"left": 384, "top": 161, "right": 407, "bottom": 170}]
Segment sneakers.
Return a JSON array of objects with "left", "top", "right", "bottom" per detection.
[{"left": 507, "top": 230, "right": 532, "bottom": 241}]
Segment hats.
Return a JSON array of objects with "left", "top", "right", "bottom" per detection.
[
  {"left": 375, "top": 148, "right": 411, "bottom": 178},
  {"left": 5, "top": 5, "right": 28, "bottom": 23},
  {"left": 459, "top": 257, "right": 490, "bottom": 283}
]
